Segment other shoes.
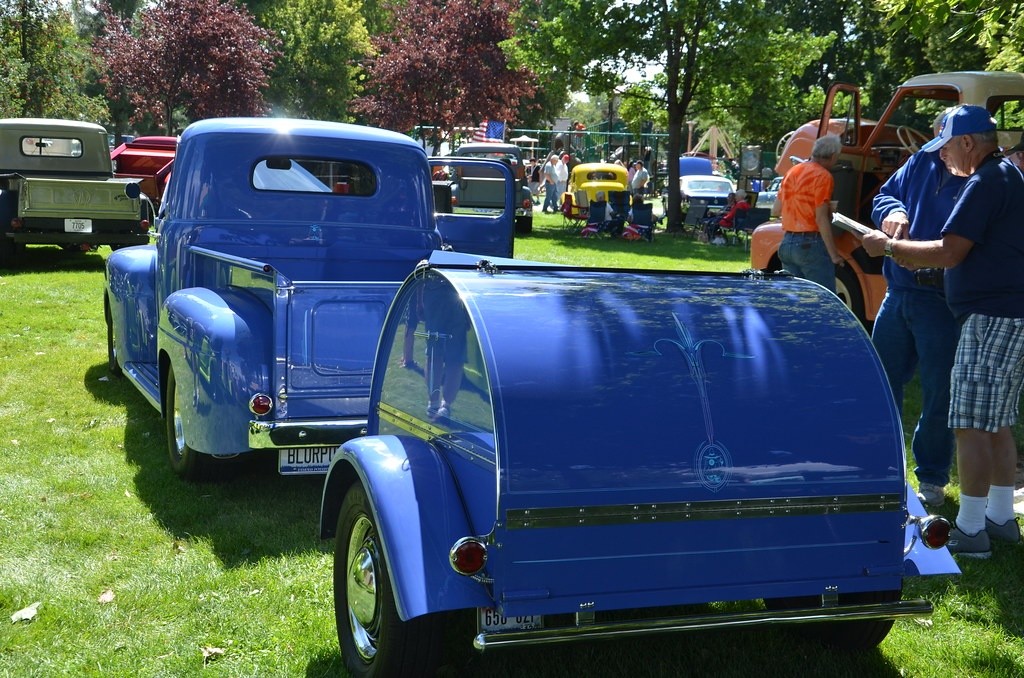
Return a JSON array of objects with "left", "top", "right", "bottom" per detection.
[
  {"left": 712, "top": 237, "right": 726, "bottom": 245},
  {"left": 554, "top": 209, "right": 559, "bottom": 212},
  {"left": 542, "top": 208, "right": 548, "bottom": 212},
  {"left": 534, "top": 201, "right": 540, "bottom": 205}
]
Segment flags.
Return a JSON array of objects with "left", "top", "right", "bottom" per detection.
[{"left": 472, "top": 119, "right": 504, "bottom": 143}]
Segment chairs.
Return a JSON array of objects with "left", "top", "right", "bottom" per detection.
[
  {"left": 741, "top": 207, "right": 772, "bottom": 252},
  {"left": 580, "top": 199, "right": 611, "bottom": 240},
  {"left": 672, "top": 202, "right": 708, "bottom": 240},
  {"left": 560, "top": 194, "right": 587, "bottom": 233},
  {"left": 629, "top": 201, "right": 655, "bottom": 243},
  {"left": 732, "top": 207, "right": 747, "bottom": 245},
  {"left": 690, "top": 207, "right": 731, "bottom": 242},
  {"left": 608, "top": 190, "right": 630, "bottom": 237}
]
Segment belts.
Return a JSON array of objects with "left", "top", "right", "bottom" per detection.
[{"left": 786, "top": 230, "right": 821, "bottom": 238}]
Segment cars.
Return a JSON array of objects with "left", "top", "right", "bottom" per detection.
[
  {"left": 561, "top": 162, "right": 633, "bottom": 218},
  {"left": 755, "top": 176, "right": 783, "bottom": 209},
  {"left": 661, "top": 158, "right": 735, "bottom": 219}
]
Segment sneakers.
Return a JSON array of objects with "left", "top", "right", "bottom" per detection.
[
  {"left": 946, "top": 521, "right": 993, "bottom": 559},
  {"left": 917, "top": 483, "right": 946, "bottom": 507},
  {"left": 985, "top": 516, "right": 1021, "bottom": 544}
]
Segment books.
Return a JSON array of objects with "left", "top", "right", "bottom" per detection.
[{"left": 831, "top": 211, "right": 874, "bottom": 240}]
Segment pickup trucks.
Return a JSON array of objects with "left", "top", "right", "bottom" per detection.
[
  {"left": 104, "top": 118, "right": 516, "bottom": 481},
  {"left": 0, "top": 118, "right": 156, "bottom": 266},
  {"left": 449, "top": 143, "right": 532, "bottom": 231},
  {"left": 111, "top": 135, "right": 178, "bottom": 213}
]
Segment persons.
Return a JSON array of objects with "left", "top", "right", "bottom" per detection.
[
  {"left": 553, "top": 154, "right": 568, "bottom": 208},
  {"left": 168, "top": 299, "right": 205, "bottom": 413},
  {"left": 627, "top": 194, "right": 658, "bottom": 226},
  {"left": 851, "top": 103, "right": 1024, "bottom": 559},
  {"left": 704, "top": 189, "right": 750, "bottom": 245},
  {"left": 542, "top": 155, "right": 559, "bottom": 212},
  {"left": 595, "top": 191, "right": 615, "bottom": 221},
  {"left": 573, "top": 120, "right": 585, "bottom": 145},
  {"left": 772, "top": 134, "right": 844, "bottom": 295},
  {"left": 625, "top": 158, "right": 635, "bottom": 195},
  {"left": 398, "top": 273, "right": 472, "bottom": 422},
  {"left": 528, "top": 158, "right": 541, "bottom": 204},
  {"left": 632, "top": 159, "right": 650, "bottom": 204}
]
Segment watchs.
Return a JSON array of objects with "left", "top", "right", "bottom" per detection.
[{"left": 884, "top": 239, "right": 894, "bottom": 258}]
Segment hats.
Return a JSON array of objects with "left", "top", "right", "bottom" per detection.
[{"left": 922, "top": 105, "right": 997, "bottom": 153}]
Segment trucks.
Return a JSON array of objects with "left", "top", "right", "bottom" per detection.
[{"left": 749, "top": 71, "right": 1024, "bottom": 321}]
